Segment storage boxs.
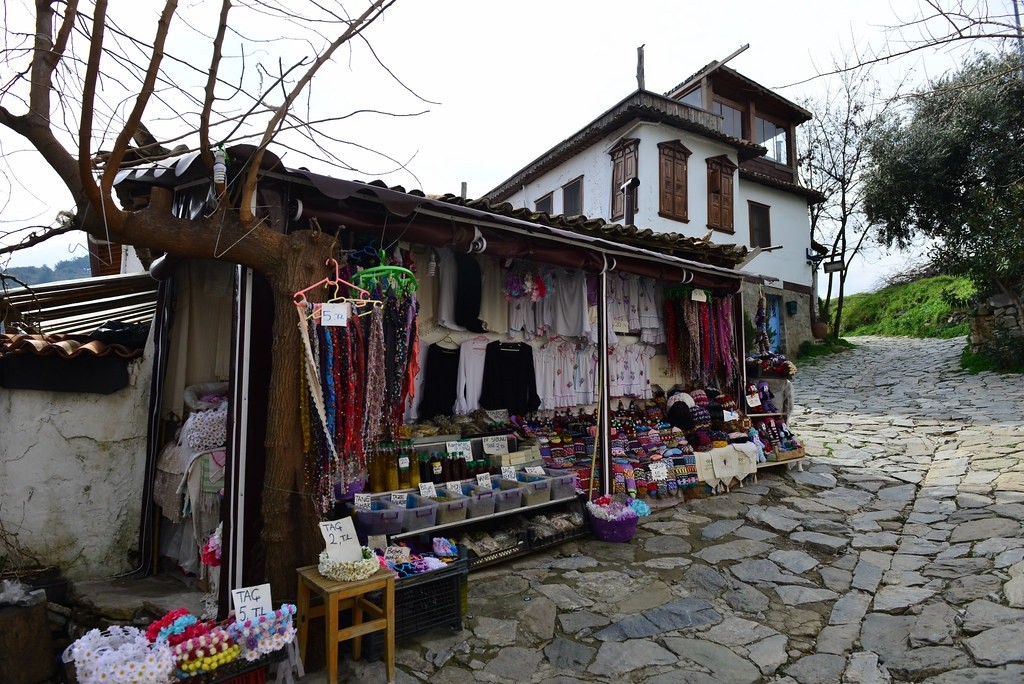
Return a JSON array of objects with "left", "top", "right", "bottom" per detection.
[
  {"left": 167, "top": 646, "right": 289, "bottom": 684},
  {"left": 335, "top": 465, "right": 640, "bottom": 664}
]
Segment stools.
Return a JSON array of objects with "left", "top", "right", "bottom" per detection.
[{"left": 294, "top": 563, "right": 396, "bottom": 683}]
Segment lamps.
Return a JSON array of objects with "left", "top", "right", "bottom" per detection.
[
  {"left": 212, "top": 144, "right": 227, "bottom": 184},
  {"left": 426, "top": 250, "right": 437, "bottom": 278}
]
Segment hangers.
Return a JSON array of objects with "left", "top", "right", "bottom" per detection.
[
  {"left": 294, "top": 258, "right": 371, "bottom": 307},
  {"left": 297, "top": 280, "right": 383, "bottom": 329},
  {"left": 347, "top": 250, "right": 419, "bottom": 303}
]
